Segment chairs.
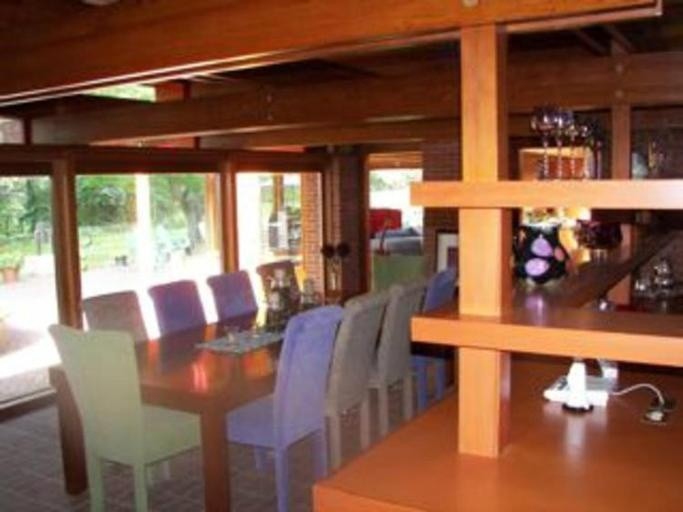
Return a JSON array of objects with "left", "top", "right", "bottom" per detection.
[
  {"left": 81, "top": 291, "right": 150, "bottom": 345},
  {"left": 410, "top": 267, "right": 458, "bottom": 414},
  {"left": 206, "top": 270, "right": 258, "bottom": 322},
  {"left": 317, "top": 288, "right": 391, "bottom": 473},
  {"left": 225, "top": 304, "right": 346, "bottom": 509},
  {"left": 357, "top": 278, "right": 425, "bottom": 451},
  {"left": 52, "top": 324, "right": 201, "bottom": 510},
  {"left": 258, "top": 260, "right": 430, "bottom": 311},
  {"left": 148, "top": 279, "right": 208, "bottom": 335}
]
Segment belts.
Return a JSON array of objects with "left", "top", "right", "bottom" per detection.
[{"left": 530, "top": 99, "right": 596, "bottom": 179}]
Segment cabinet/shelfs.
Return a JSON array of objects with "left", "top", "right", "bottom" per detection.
[{"left": 270, "top": 269, "right": 290, "bottom": 327}]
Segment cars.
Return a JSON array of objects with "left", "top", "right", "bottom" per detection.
[
  {"left": 224, "top": 324, "right": 241, "bottom": 346},
  {"left": 248, "top": 322, "right": 260, "bottom": 340}
]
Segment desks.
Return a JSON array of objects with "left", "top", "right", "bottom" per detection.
[{"left": 52, "top": 294, "right": 357, "bottom": 510}]
